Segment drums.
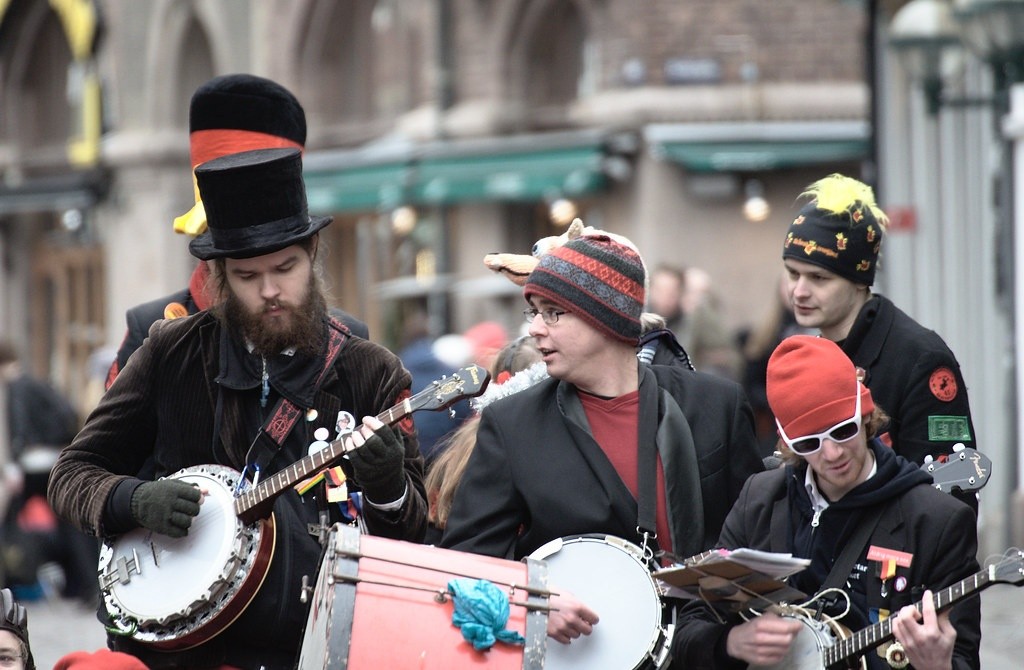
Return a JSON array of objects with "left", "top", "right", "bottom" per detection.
[
  {"left": 528, "top": 533, "right": 676, "bottom": 670},
  {"left": 297, "top": 522, "right": 559, "bottom": 670}
]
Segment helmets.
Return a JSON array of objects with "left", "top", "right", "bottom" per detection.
[{"left": 0, "top": 587, "right": 34, "bottom": 669}]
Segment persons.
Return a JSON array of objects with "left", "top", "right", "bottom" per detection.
[
  {"left": 482, "top": 217, "right": 697, "bottom": 379},
  {"left": 434, "top": 232, "right": 766, "bottom": 643},
  {"left": 99, "top": 73, "right": 371, "bottom": 396},
  {"left": 0, "top": 588, "right": 37, "bottom": 670},
  {"left": 654, "top": 334, "right": 984, "bottom": 670},
  {"left": 0, "top": 339, "right": 107, "bottom": 610},
  {"left": 39, "top": 143, "right": 430, "bottom": 670},
  {"left": 780, "top": 170, "right": 981, "bottom": 522},
  {"left": 650, "top": 267, "right": 747, "bottom": 387},
  {"left": 397, "top": 323, "right": 548, "bottom": 525}
]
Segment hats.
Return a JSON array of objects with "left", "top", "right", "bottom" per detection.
[
  {"left": 483, "top": 215, "right": 664, "bottom": 337},
  {"left": 766, "top": 335, "right": 874, "bottom": 448},
  {"left": 783, "top": 173, "right": 888, "bottom": 287},
  {"left": 466, "top": 323, "right": 507, "bottom": 372},
  {"left": 524, "top": 234, "right": 643, "bottom": 345},
  {"left": 190, "top": 148, "right": 334, "bottom": 261},
  {"left": 172, "top": 74, "right": 307, "bottom": 235}
]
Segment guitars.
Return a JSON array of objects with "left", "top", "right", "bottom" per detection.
[
  {"left": 747, "top": 550, "right": 1024, "bottom": 670},
  {"left": 96, "top": 362, "right": 492, "bottom": 651},
  {"left": 921, "top": 443, "right": 993, "bottom": 495}
]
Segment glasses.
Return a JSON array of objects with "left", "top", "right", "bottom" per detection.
[
  {"left": 776, "top": 377, "right": 862, "bottom": 455},
  {"left": 509, "top": 336, "right": 531, "bottom": 373},
  {"left": 522, "top": 308, "right": 570, "bottom": 325}
]
